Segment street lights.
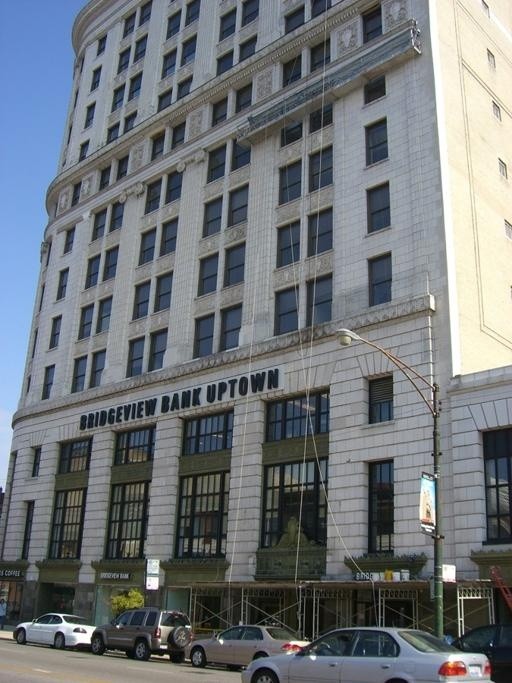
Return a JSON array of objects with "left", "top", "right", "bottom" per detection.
[{"left": 334, "top": 326, "right": 446, "bottom": 637}]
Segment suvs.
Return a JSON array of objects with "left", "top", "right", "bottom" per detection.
[{"left": 89, "top": 606, "right": 193, "bottom": 665}]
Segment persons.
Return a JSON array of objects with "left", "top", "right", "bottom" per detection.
[{"left": 0, "top": 598, "right": 8, "bottom": 630}]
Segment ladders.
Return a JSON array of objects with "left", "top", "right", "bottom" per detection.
[{"left": 489, "top": 566, "right": 512, "bottom": 610}]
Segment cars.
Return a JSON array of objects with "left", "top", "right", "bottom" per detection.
[
  {"left": 240, "top": 624, "right": 493, "bottom": 683},
  {"left": 183, "top": 621, "right": 317, "bottom": 670},
  {"left": 12, "top": 611, "right": 98, "bottom": 650},
  {"left": 445, "top": 622, "right": 511, "bottom": 682}
]
